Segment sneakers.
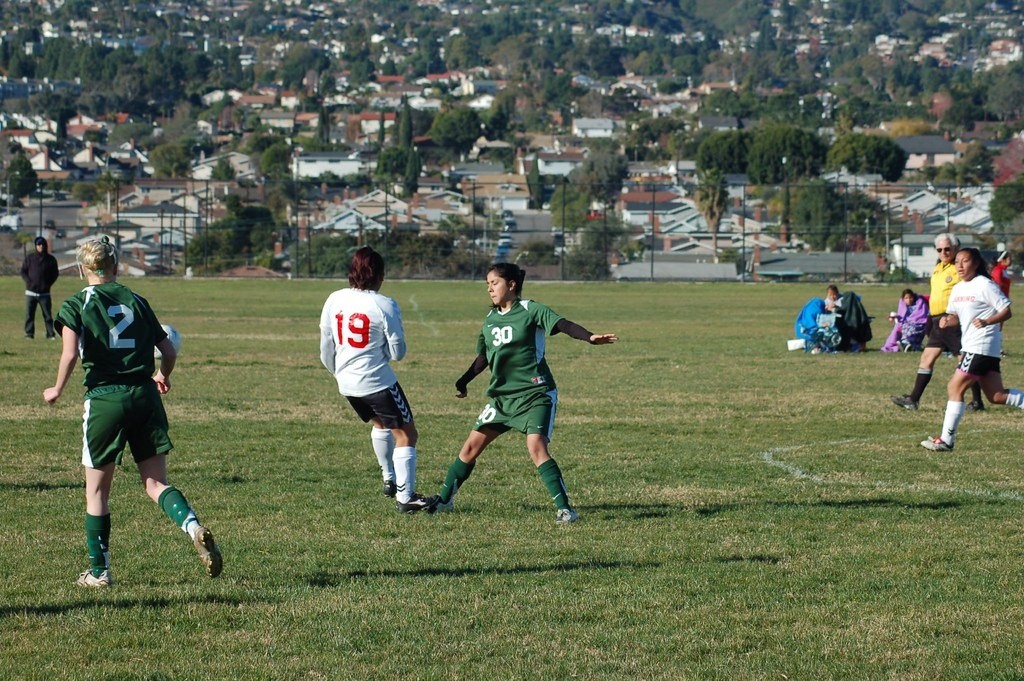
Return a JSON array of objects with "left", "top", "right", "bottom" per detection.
[
  {"left": 435, "top": 499, "right": 454, "bottom": 511},
  {"left": 889, "top": 396, "right": 919, "bottom": 410},
  {"left": 921, "top": 435, "right": 954, "bottom": 451},
  {"left": 395, "top": 493, "right": 441, "bottom": 514},
  {"left": 77, "top": 570, "right": 109, "bottom": 587},
  {"left": 383, "top": 480, "right": 397, "bottom": 498},
  {"left": 194, "top": 526, "right": 223, "bottom": 579},
  {"left": 555, "top": 508, "right": 577, "bottom": 524},
  {"left": 965, "top": 400, "right": 986, "bottom": 412}
]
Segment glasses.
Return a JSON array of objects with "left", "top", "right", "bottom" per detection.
[{"left": 936, "top": 246, "right": 955, "bottom": 253}]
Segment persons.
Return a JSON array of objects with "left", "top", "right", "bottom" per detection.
[
  {"left": 888, "top": 288, "right": 929, "bottom": 352},
  {"left": 319, "top": 246, "right": 441, "bottom": 516},
  {"left": 43, "top": 241, "right": 224, "bottom": 588},
  {"left": 890, "top": 233, "right": 983, "bottom": 411},
  {"left": 990, "top": 251, "right": 1013, "bottom": 356},
  {"left": 794, "top": 285, "right": 873, "bottom": 355},
  {"left": 21, "top": 236, "right": 56, "bottom": 340},
  {"left": 920, "top": 247, "right": 1024, "bottom": 450},
  {"left": 426, "top": 263, "right": 619, "bottom": 524}
]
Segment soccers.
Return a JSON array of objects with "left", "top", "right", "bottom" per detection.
[{"left": 156, "top": 324, "right": 181, "bottom": 358}]
